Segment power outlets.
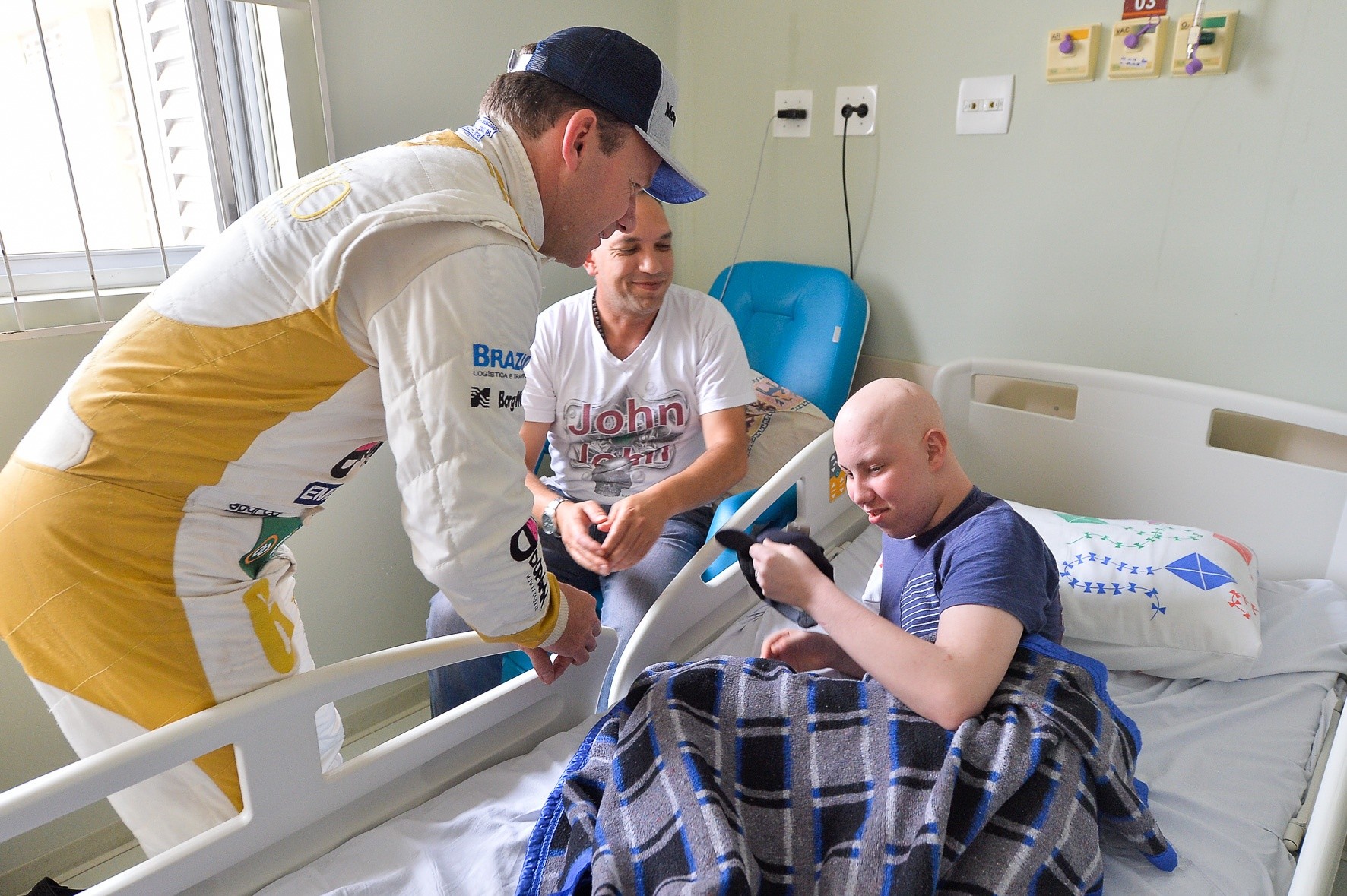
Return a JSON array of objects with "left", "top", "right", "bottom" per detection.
[
  {"left": 769, "top": 89, "right": 818, "bottom": 141},
  {"left": 834, "top": 88, "right": 879, "bottom": 138},
  {"left": 953, "top": 74, "right": 1016, "bottom": 136}
]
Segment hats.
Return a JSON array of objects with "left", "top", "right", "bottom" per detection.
[
  {"left": 507, "top": 26, "right": 708, "bottom": 206},
  {"left": 712, "top": 527, "right": 833, "bottom": 629}
]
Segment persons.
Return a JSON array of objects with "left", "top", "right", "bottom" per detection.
[
  {"left": 757, "top": 375, "right": 1067, "bottom": 732},
  {"left": 427, "top": 187, "right": 754, "bottom": 730},
  {"left": 1, "top": 23, "right": 712, "bottom": 873}
]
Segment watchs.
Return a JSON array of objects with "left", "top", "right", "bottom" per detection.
[{"left": 541, "top": 496, "right": 568, "bottom": 538}]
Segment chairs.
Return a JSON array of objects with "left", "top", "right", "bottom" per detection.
[{"left": 493, "top": 258, "right": 872, "bottom": 678}]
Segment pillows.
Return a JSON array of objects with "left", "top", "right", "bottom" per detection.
[{"left": 999, "top": 497, "right": 1266, "bottom": 686}]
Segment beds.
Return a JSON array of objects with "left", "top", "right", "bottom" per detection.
[{"left": 0, "top": 350, "right": 1347, "bottom": 896}]
